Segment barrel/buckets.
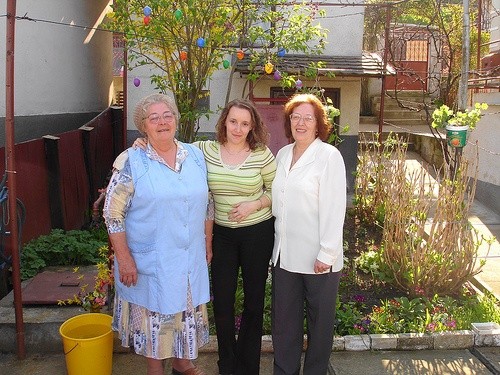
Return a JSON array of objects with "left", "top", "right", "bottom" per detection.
[{"left": 58, "top": 312, "right": 114, "bottom": 375}]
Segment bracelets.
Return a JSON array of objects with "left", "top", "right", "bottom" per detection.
[{"left": 257, "top": 198, "right": 263, "bottom": 212}]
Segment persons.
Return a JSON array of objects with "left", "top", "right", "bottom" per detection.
[
  {"left": 133, "top": 100, "right": 277, "bottom": 375},
  {"left": 270, "top": 94, "right": 347, "bottom": 375},
  {"left": 102, "top": 94, "right": 215, "bottom": 375}
]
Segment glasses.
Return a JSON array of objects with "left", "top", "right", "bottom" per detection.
[
  {"left": 289, "top": 112, "right": 318, "bottom": 125},
  {"left": 142, "top": 112, "right": 176, "bottom": 124}
]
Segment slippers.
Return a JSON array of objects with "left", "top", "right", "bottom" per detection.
[{"left": 171, "top": 365, "right": 205, "bottom": 375}]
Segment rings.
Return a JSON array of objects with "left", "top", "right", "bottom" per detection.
[{"left": 136, "top": 142, "right": 138, "bottom": 144}]
